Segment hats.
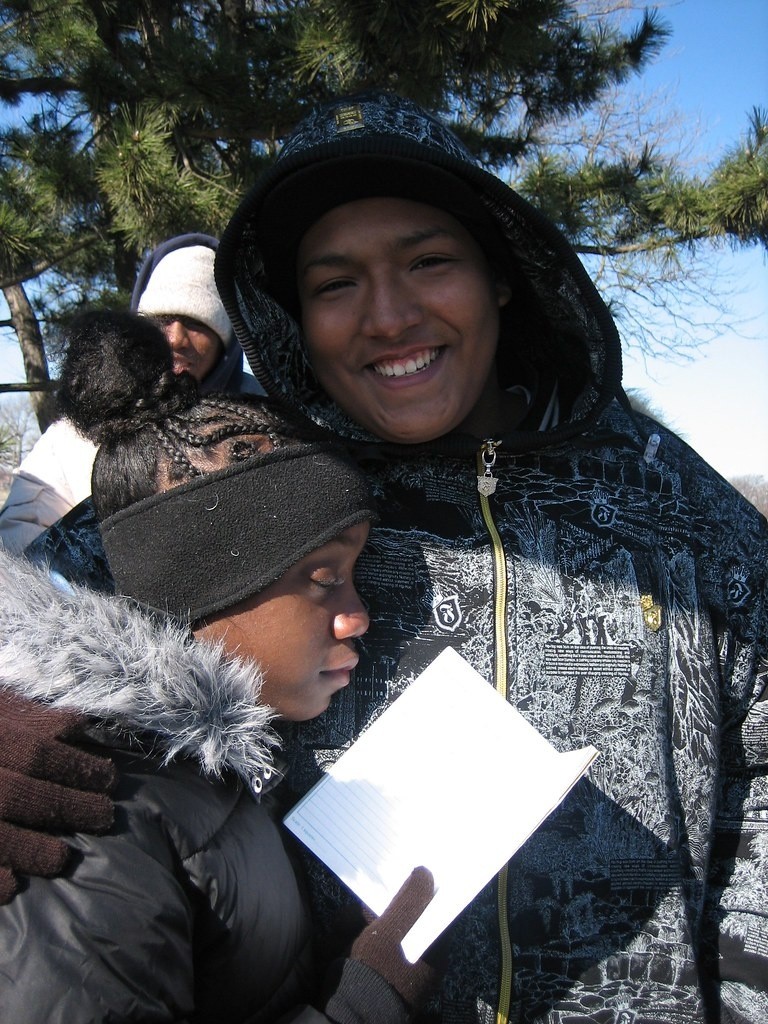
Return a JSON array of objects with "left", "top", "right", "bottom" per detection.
[
  {"left": 261, "top": 154, "right": 527, "bottom": 334},
  {"left": 137, "top": 246, "right": 234, "bottom": 347}
]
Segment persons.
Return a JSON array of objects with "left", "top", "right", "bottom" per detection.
[
  {"left": 0, "top": 106, "right": 768, "bottom": 1023},
  {"left": 1, "top": 231, "right": 283, "bottom": 561},
  {"left": 0, "top": 288, "right": 455, "bottom": 1024}
]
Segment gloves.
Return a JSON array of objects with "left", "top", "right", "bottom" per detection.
[
  {"left": 0, "top": 685, "right": 119, "bottom": 906},
  {"left": 350, "top": 866, "right": 447, "bottom": 1015}
]
